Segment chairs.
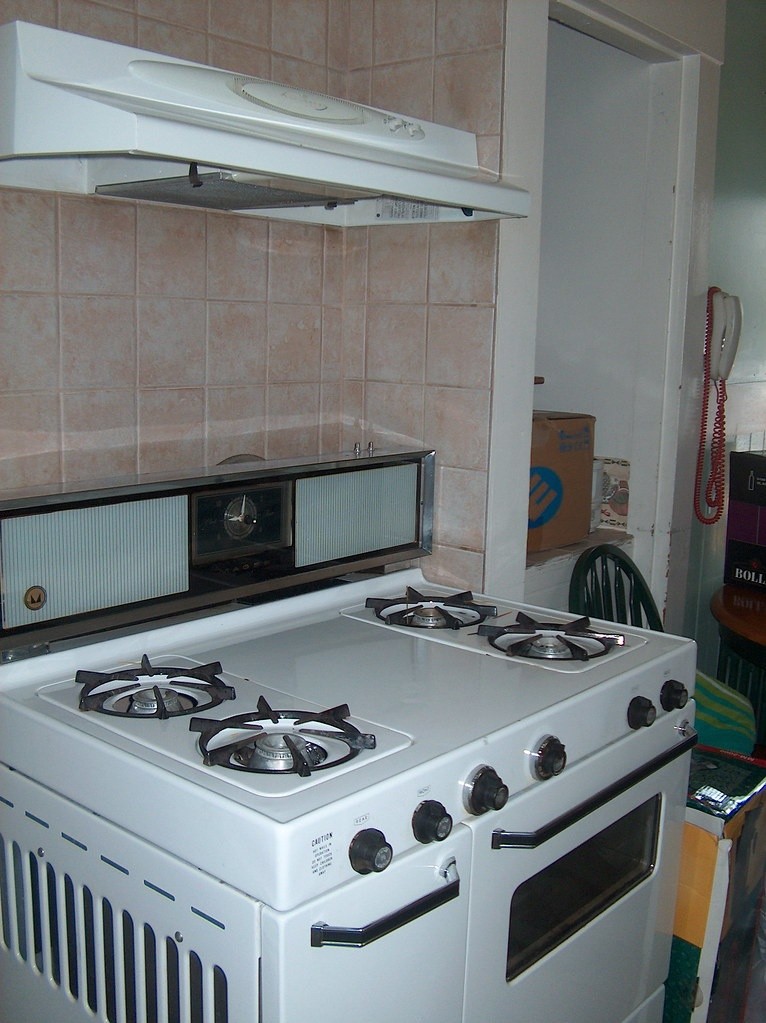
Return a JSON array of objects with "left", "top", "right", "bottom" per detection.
[{"left": 565, "top": 543, "right": 664, "bottom": 634}]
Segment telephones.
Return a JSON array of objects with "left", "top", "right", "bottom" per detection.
[{"left": 708, "top": 292, "right": 742, "bottom": 382}]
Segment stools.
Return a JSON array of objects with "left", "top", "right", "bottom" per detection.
[{"left": 709, "top": 582, "right": 765, "bottom": 724}]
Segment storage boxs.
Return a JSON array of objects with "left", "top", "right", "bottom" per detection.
[
  {"left": 525, "top": 406, "right": 597, "bottom": 556},
  {"left": 722, "top": 450, "right": 766, "bottom": 592}
]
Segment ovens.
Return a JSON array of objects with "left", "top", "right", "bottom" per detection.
[{"left": 462, "top": 694, "right": 697, "bottom": 1021}]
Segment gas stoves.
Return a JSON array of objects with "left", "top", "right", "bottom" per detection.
[{"left": 2, "top": 566, "right": 698, "bottom": 910}]
